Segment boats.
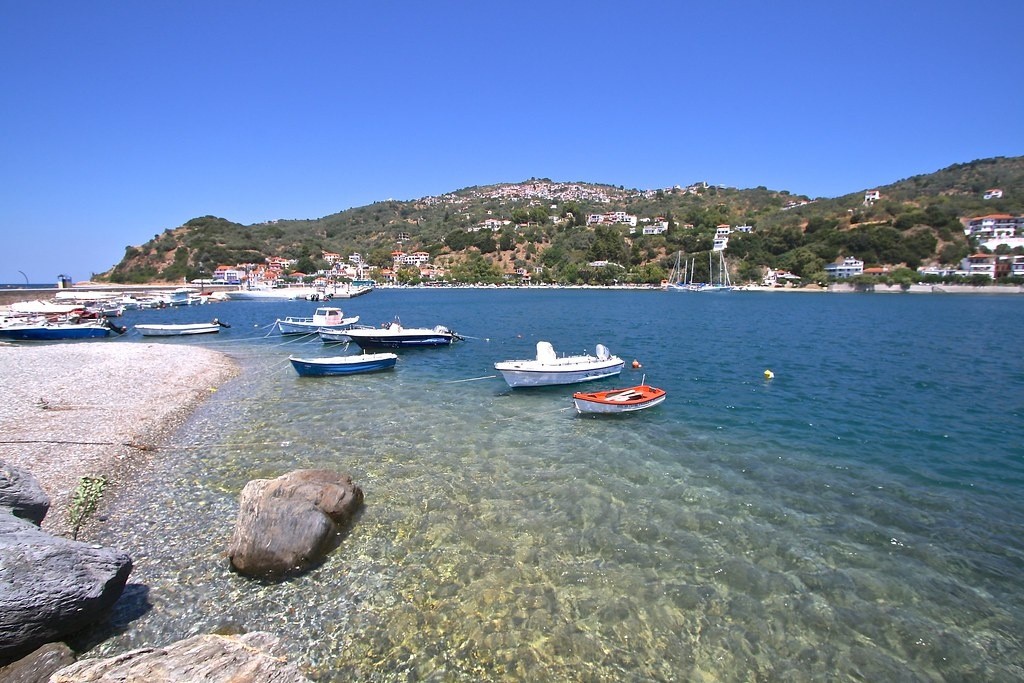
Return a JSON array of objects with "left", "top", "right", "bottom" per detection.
[
  {"left": 493, "top": 339, "right": 626, "bottom": 388},
  {"left": 0, "top": 316, "right": 110, "bottom": 340},
  {"left": 288, "top": 354, "right": 397, "bottom": 376},
  {"left": 572, "top": 383, "right": 666, "bottom": 415},
  {"left": 278, "top": 307, "right": 359, "bottom": 334},
  {"left": 318, "top": 327, "right": 353, "bottom": 345},
  {"left": 6, "top": 287, "right": 209, "bottom": 320},
  {"left": 134, "top": 321, "right": 221, "bottom": 336},
  {"left": 346, "top": 323, "right": 453, "bottom": 350}
]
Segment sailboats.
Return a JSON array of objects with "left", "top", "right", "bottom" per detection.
[{"left": 666, "top": 250, "right": 736, "bottom": 294}]
